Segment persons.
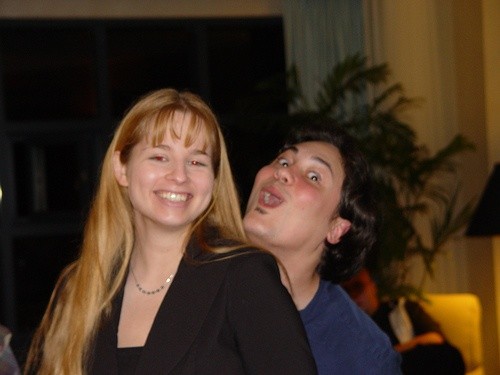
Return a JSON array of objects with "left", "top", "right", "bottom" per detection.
[
  {"left": 342, "top": 265, "right": 466, "bottom": 375},
  {"left": 239, "top": 122, "right": 403, "bottom": 375},
  {"left": 24, "top": 87, "right": 320, "bottom": 375}
]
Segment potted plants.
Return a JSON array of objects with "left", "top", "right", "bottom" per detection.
[{"left": 274, "top": 55, "right": 481, "bottom": 344}]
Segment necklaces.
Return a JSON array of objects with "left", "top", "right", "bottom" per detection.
[{"left": 128, "top": 261, "right": 175, "bottom": 294}]
